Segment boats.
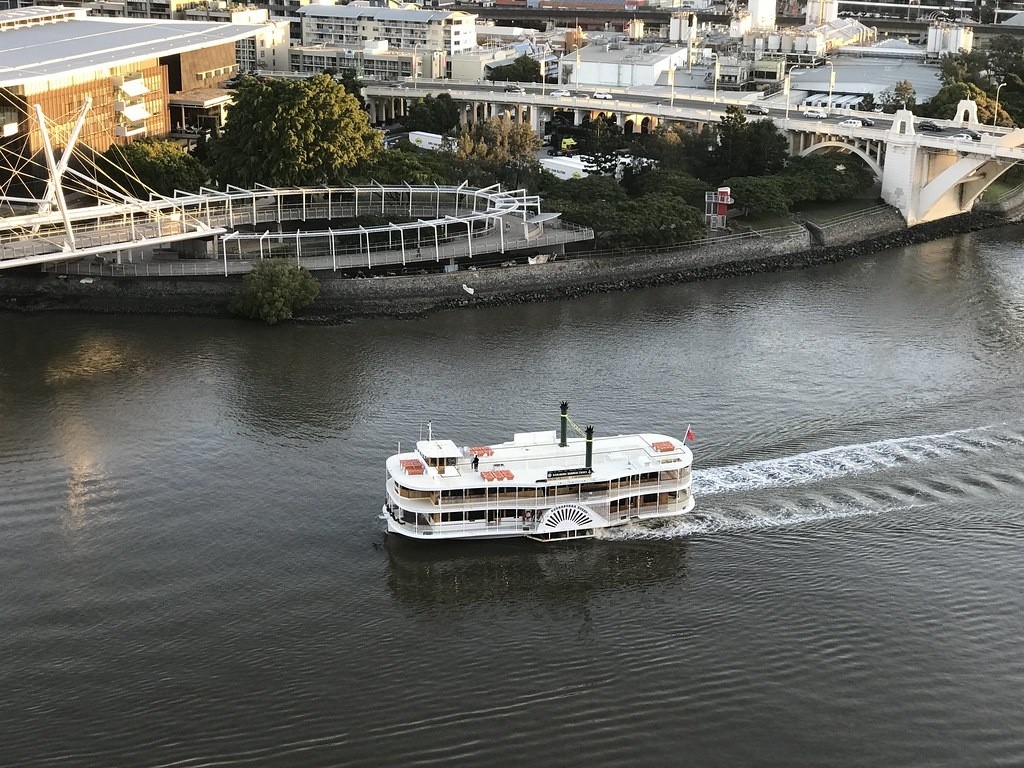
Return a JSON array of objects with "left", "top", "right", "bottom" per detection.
[{"left": 377, "top": 400, "right": 696, "bottom": 542}]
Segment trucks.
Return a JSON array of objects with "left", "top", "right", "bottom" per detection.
[{"left": 541, "top": 134, "right": 558, "bottom": 148}]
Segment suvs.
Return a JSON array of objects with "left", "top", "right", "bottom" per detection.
[{"left": 918, "top": 120, "right": 944, "bottom": 132}]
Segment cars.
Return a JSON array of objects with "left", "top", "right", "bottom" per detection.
[
  {"left": 746, "top": 102, "right": 770, "bottom": 115},
  {"left": 803, "top": 108, "right": 828, "bottom": 119},
  {"left": 388, "top": 84, "right": 402, "bottom": 90},
  {"left": 546, "top": 147, "right": 563, "bottom": 156},
  {"left": 852, "top": 116, "right": 875, "bottom": 126},
  {"left": 504, "top": 84, "right": 526, "bottom": 94},
  {"left": 549, "top": 89, "right": 571, "bottom": 97},
  {"left": 573, "top": 93, "right": 590, "bottom": 100},
  {"left": 948, "top": 133, "right": 973, "bottom": 141},
  {"left": 592, "top": 92, "right": 613, "bottom": 100},
  {"left": 958, "top": 128, "right": 982, "bottom": 140},
  {"left": 258, "top": 75, "right": 271, "bottom": 82},
  {"left": 839, "top": 119, "right": 863, "bottom": 128},
  {"left": 374, "top": 127, "right": 391, "bottom": 136}
]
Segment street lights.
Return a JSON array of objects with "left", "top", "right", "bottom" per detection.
[
  {"left": 542, "top": 48, "right": 553, "bottom": 96},
  {"left": 414, "top": 43, "right": 420, "bottom": 88},
  {"left": 825, "top": 61, "right": 834, "bottom": 113},
  {"left": 991, "top": 82, "right": 1007, "bottom": 158},
  {"left": 785, "top": 64, "right": 800, "bottom": 129},
  {"left": 708, "top": 52, "right": 718, "bottom": 105},
  {"left": 572, "top": 43, "right": 579, "bottom": 93}
]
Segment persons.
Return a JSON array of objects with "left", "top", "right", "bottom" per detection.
[
  {"left": 477, "top": 78, "right": 480, "bottom": 85},
  {"left": 516, "top": 81, "right": 519, "bottom": 85},
  {"left": 470, "top": 455, "right": 479, "bottom": 472},
  {"left": 506, "top": 222, "right": 510, "bottom": 228}
]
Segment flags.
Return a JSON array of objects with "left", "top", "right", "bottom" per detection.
[{"left": 686, "top": 424, "right": 695, "bottom": 441}]
